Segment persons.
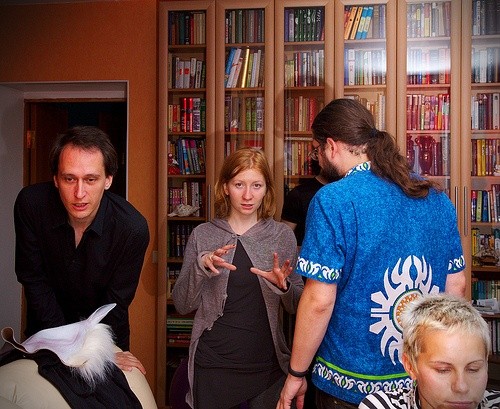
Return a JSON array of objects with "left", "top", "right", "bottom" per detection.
[
  {"left": 358, "top": 294, "right": 500, "bottom": 409},
  {"left": 173, "top": 148, "right": 340, "bottom": 409},
  {"left": 13, "top": 126, "right": 146, "bottom": 375},
  {"left": 276, "top": 99, "right": 466, "bottom": 409}
]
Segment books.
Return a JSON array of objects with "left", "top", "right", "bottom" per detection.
[{"left": 168, "top": 1, "right": 500, "bottom": 359}]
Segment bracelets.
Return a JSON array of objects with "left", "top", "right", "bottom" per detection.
[{"left": 288, "top": 363, "right": 308, "bottom": 378}]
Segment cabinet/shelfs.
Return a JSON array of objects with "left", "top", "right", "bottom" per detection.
[{"left": 158, "top": 0, "right": 500, "bottom": 409}]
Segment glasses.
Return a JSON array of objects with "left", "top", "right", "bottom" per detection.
[{"left": 309, "top": 137, "right": 338, "bottom": 160}]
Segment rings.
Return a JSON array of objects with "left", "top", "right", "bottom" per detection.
[{"left": 210, "top": 255, "right": 214, "bottom": 261}]
